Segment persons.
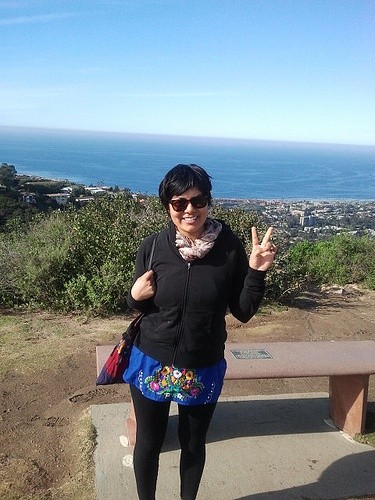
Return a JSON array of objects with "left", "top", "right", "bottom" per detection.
[{"left": 96, "top": 164, "right": 276, "bottom": 500}]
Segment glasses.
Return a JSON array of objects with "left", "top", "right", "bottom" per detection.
[{"left": 167, "top": 194, "right": 211, "bottom": 212}]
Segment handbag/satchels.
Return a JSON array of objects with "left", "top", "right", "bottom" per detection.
[{"left": 94, "top": 313, "right": 143, "bottom": 386}]
